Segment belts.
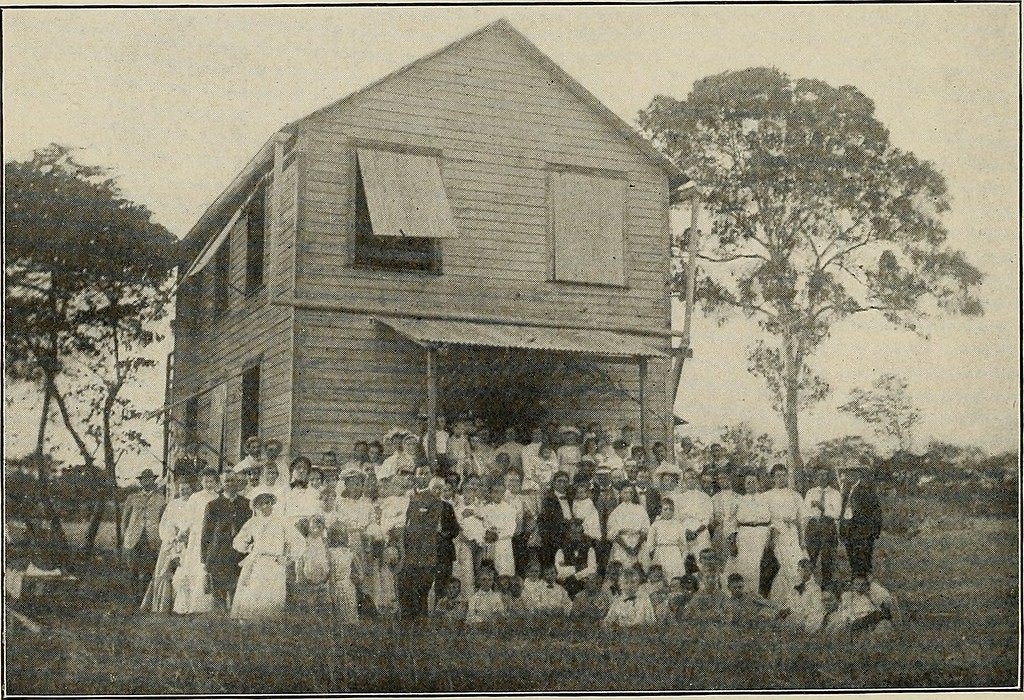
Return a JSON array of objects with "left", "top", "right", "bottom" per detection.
[{"left": 739, "top": 521, "right": 772, "bottom": 527}]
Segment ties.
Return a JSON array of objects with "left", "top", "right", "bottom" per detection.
[{"left": 819, "top": 490, "right": 825, "bottom": 516}]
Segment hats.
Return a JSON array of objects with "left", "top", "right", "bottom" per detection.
[
  {"left": 559, "top": 425, "right": 582, "bottom": 437},
  {"left": 263, "top": 438, "right": 284, "bottom": 451},
  {"left": 401, "top": 433, "right": 418, "bottom": 446},
  {"left": 653, "top": 459, "right": 683, "bottom": 489},
  {"left": 248, "top": 488, "right": 278, "bottom": 510},
  {"left": 382, "top": 426, "right": 412, "bottom": 443},
  {"left": 381, "top": 540, "right": 407, "bottom": 576},
  {"left": 836, "top": 458, "right": 873, "bottom": 474},
  {"left": 243, "top": 436, "right": 265, "bottom": 455},
  {"left": 338, "top": 468, "right": 368, "bottom": 482},
  {"left": 136, "top": 468, "right": 158, "bottom": 480},
  {"left": 288, "top": 454, "right": 313, "bottom": 476}
]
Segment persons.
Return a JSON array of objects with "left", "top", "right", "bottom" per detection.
[{"left": 118, "top": 415, "right": 900, "bottom": 637}]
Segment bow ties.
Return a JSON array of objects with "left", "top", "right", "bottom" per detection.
[
  {"left": 609, "top": 582, "right": 622, "bottom": 596},
  {"left": 793, "top": 582, "right": 806, "bottom": 595},
  {"left": 624, "top": 594, "right": 636, "bottom": 601},
  {"left": 638, "top": 491, "right": 647, "bottom": 496},
  {"left": 558, "top": 495, "right": 568, "bottom": 501},
  {"left": 545, "top": 584, "right": 555, "bottom": 589}
]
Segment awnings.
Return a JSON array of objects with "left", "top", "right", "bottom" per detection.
[
  {"left": 143, "top": 364, "right": 251, "bottom": 473},
  {"left": 148, "top": 185, "right": 259, "bottom": 318},
  {"left": 367, "top": 312, "right": 675, "bottom": 464}
]
